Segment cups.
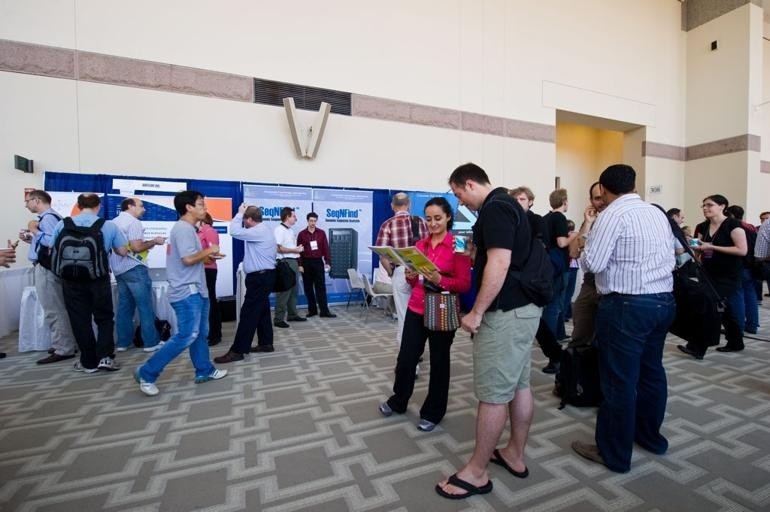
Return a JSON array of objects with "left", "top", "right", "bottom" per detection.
[
  {"left": 454, "top": 235, "right": 466, "bottom": 254},
  {"left": 689, "top": 239, "right": 699, "bottom": 247}
]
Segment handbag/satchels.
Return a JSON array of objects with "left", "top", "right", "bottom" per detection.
[
  {"left": 37, "top": 213, "right": 60, "bottom": 271},
  {"left": 667, "top": 271, "right": 727, "bottom": 346},
  {"left": 276, "top": 260, "right": 297, "bottom": 291},
  {"left": 552, "top": 336, "right": 602, "bottom": 410},
  {"left": 423, "top": 292, "right": 461, "bottom": 333},
  {"left": 132, "top": 317, "right": 171, "bottom": 347}
]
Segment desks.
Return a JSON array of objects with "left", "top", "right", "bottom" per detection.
[
  {"left": 20, "top": 281, "right": 179, "bottom": 352},
  {"left": 2, "top": 261, "right": 36, "bottom": 336}
]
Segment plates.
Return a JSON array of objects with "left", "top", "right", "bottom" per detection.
[{"left": 212, "top": 255, "right": 224, "bottom": 259}]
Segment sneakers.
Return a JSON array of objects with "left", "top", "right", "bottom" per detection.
[
  {"left": 135, "top": 367, "right": 159, "bottom": 396},
  {"left": 72, "top": 359, "right": 99, "bottom": 374},
  {"left": 116, "top": 346, "right": 128, "bottom": 352},
  {"left": 143, "top": 340, "right": 165, "bottom": 352},
  {"left": 97, "top": 356, "right": 120, "bottom": 371},
  {"left": 377, "top": 402, "right": 392, "bottom": 416},
  {"left": 195, "top": 369, "right": 228, "bottom": 383},
  {"left": 417, "top": 418, "right": 436, "bottom": 432}
]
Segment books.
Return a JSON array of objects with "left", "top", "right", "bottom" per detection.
[{"left": 365, "top": 244, "right": 440, "bottom": 278}]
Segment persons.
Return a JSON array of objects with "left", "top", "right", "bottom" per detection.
[
  {"left": 133, "top": 191, "right": 227, "bottom": 396},
  {"left": 511, "top": 187, "right": 576, "bottom": 373},
  {"left": 435, "top": 162, "right": 545, "bottom": 499},
  {"left": 571, "top": 180, "right": 608, "bottom": 411},
  {"left": 50, "top": 193, "right": 129, "bottom": 372},
  {"left": 668, "top": 194, "right": 769, "bottom": 360},
  {"left": 195, "top": 212, "right": 222, "bottom": 347},
  {"left": 571, "top": 162, "right": 669, "bottom": 473},
  {"left": 109, "top": 196, "right": 166, "bottom": 353},
  {"left": 375, "top": 191, "right": 431, "bottom": 381},
  {"left": 215, "top": 202, "right": 277, "bottom": 363},
  {"left": 375, "top": 196, "right": 472, "bottom": 431},
  {"left": 297, "top": 213, "right": 338, "bottom": 316},
  {"left": 273, "top": 207, "right": 307, "bottom": 328},
  {"left": 0, "top": 189, "right": 78, "bottom": 365}
]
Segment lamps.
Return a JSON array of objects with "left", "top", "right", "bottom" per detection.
[{"left": 282, "top": 96, "right": 333, "bottom": 162}]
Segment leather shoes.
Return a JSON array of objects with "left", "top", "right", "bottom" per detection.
[
  {"left": 320, "top": 311, "right": 336, "bottom": 317},
  {"left": 542, "top": 361, "right": 560, "bottom": 373},
  {"left": 36, "top": 353, "right": 75, "bottom": 364},
  {"left": 274, "top": 320, "right": 289, "bottom": 328},
  {"left": 250, "top": 345, "right": 273, "bottom": 352},
  {"left": 208, "top": 337, "right": 221, "bottom": 346},
  {"left": 678, "top": 345, "right": 703, "bottom": 359},
  {"left": 720, "top": 329, "right": 725, "bottom": 334},
  {"left": 716, "top": 346, "right": 730, "bottom": 352},
  {"left": 287, "top": 316, "right": 306, "bottom": 321},
  {"left": 306, "top": 311, "right": 317, "bottom": 317},
  {"left": 571, "top": 439, "right": 605, "bottom": 467},
  {"left": 213, "top": 351, "right": 244, "bottom": 363}
]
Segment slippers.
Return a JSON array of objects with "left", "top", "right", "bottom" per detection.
[
  {"left": 435, "top": 474, "right": 492, "bottom": 499},
  {"left": 490, "top": 449, "right": 529, "bottom": 478}
]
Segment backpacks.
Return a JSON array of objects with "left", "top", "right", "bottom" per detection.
[
  {"left": 50, "top": 218, "right": 110, "bottom": 281},
  {"left": 519, "top": 239, "right": 556, "bottom": 307}
]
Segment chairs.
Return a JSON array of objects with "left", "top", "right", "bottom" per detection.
[
  {"left": 361, "top": 272, "right": 395, "bottom": 322},
  {"left": 348, "top": 268, "right": 373, "bottom": 311}
]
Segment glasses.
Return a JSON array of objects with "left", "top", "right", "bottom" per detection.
[
  {"left": 23, "top": 198, "right": 31, "bottom": 204},
  {"left": 701, "top": 204, "right": 718, "bottom": 208}
]
